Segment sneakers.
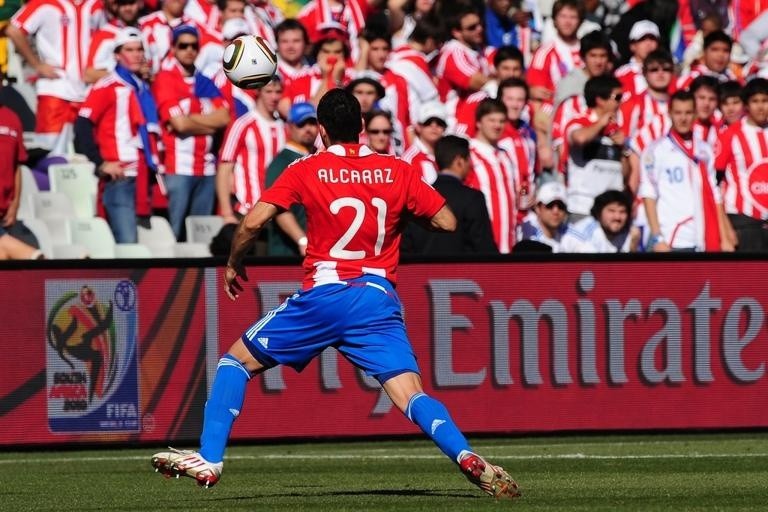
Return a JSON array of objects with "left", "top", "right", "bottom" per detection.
[
  {"left": 456, "top": 448, "right": 522, "bottom": 499},
  {"left": 150, "top": 448, "right": 225, "bottom": 491}
]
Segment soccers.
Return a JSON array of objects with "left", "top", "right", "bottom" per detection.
[{"left": 223, "top": 34, "right": 277, "bottom": 90}]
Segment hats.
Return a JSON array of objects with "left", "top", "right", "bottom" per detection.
[
  {"left": 172, "top": 24, "right": 199, "bottom": 41},
  {"left": 534, "top": 181, "right": 570, "bottom": 209},
  {"left": 286, "top": 100, "right": 317, "bottom": 125},
  {"left": 579, "top": 29, "right": 614, "bottom": 55},
  {"left": 112, "top": 26, "right": 144, "bottom": 51},
  {"left": 343, "top": 68, "right": 385, "bottom": 99},
  {"left": 417, "top": 102, "right": 449, "bottom": 125},
  {"left": 629, "top": 19, "right": 661, "bottom": 41}
]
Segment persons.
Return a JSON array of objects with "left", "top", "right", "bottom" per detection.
[
  {"left": 0, "top": 0, "right": 768, "bottom": 260},
  {"left": 152, "top": 88, "right": 520, "bottom": 497}
]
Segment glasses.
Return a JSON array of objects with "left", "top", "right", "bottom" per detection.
[
  {"left": 296, "top": 116, "right": 318, "bottom": 128},
  {"left": 421, "top": 118, "right": 446, "bottom": 127},
  {"left": 605, "top": 91, "right": 624, "bottom": 102},
  {"left": 367, "top": 128, "right": 393, "bottom": 134},
  {"left": 462, "top": 21, "right": 484, "bottom": 30},
  {"left": 645, "top": 61, "right": 674, "bottom": 73},
  {"left": 177, "top": 41, "right": 199, "bottom": 50}
]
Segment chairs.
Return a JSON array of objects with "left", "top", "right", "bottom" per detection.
[{"left": 17, "top": 165, "right": 223, "bottom": 258}]
